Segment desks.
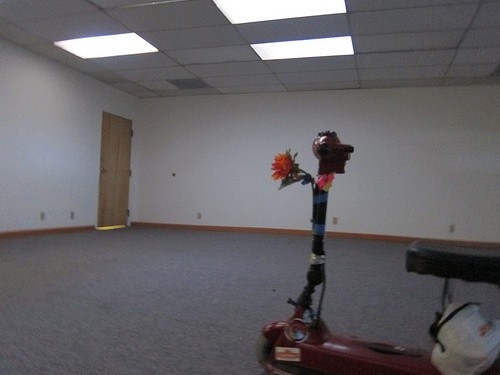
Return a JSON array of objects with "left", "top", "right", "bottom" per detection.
[{"left": 405, "top": 238, "right": 500, "bottom": 337}]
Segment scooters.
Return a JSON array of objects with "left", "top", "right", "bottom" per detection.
[{"left": 257, "top": 130, "right": 499, "bottom": 375}]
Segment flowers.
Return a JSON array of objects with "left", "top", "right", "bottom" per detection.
[{"left": 268, "top": 148, "right": 336, "bottom": 194}]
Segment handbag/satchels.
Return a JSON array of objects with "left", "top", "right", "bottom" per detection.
[{"left": 429, "top": 298, "right": 500, "bottom": 374}]
